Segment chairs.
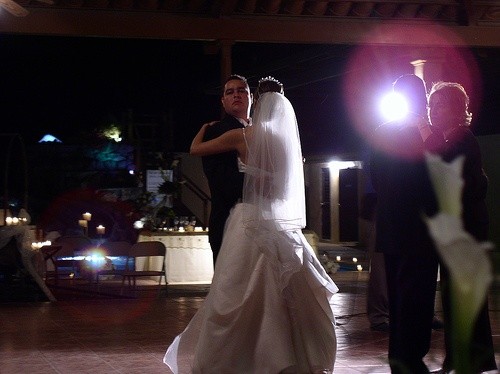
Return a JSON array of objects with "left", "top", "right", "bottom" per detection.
[{"left": 92, "top": 241, "right": 168, "bottom": 294}]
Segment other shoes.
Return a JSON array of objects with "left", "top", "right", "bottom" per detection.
[
  {"left": 378, "top": 324, "right": 390, "bottom": 331},
  {"left": 433, "top": 316, "right": 443, "bottom": 329}
]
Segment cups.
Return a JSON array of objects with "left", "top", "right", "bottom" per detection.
[{"left": 173, "top": 216, "right": 197, "bottom": 233}]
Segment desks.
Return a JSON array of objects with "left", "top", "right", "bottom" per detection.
[
  {"left": 56, "top": 257, "right": 106, "bottom": 283},
  {"left": 136, "top": 231, "right": 215, "bottom": 285}
]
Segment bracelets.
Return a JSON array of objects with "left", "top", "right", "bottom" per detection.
[{"left": 418, "top": 126, "right": 428, "bottom": 132}]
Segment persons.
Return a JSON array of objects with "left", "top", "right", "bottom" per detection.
[
  {"left": 369, "top": 74, "right": 497, "bottom": 374},
  {"left": 163, "top": 76, "right": 340, "bottom": 374},
  {"left": 201, "top": 74, "right": 253, "bottom": 272}
]
